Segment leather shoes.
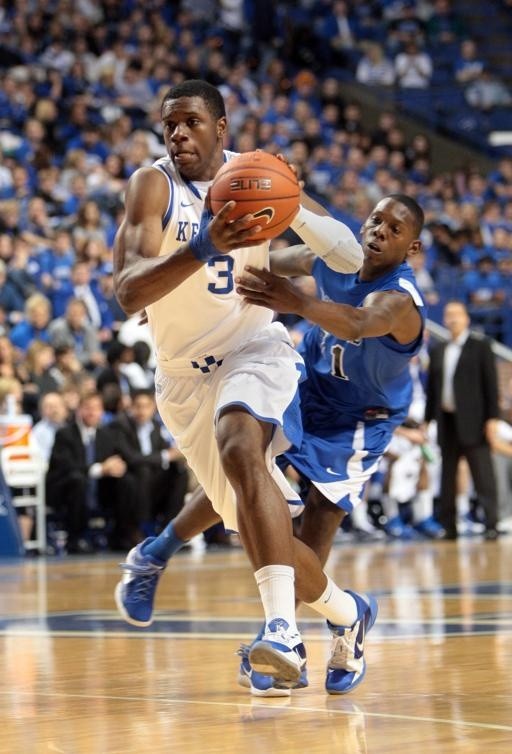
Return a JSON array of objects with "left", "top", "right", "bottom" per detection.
[
  {"left": 442, "top": 529, "right": 458, "bottom": 541},
  {"left": 482, "top": 525, "right": 497, "bottom": 542}
]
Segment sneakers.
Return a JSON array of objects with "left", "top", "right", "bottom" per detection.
[
  {"left": 323, "top": 588, "right": 379, "bottom": 697},
  {"left": 336, "top": 514, "right": 511, "bottom": 540},
  {"left": 239, "top": 618, "right": 308, "bottom": 701},
  {"left": 116, "top": 533, "right": 163, "bottom": 629}
]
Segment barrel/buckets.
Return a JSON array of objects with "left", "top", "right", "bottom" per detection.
[{"left": 0, "top": 413, "right": 35, "bottom": 474}]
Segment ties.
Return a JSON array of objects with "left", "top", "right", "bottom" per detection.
[{"left": 85, "top": 432, "right": 97, "bottom": 500}]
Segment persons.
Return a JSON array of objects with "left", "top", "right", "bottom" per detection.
[
  {"left": 114, "top": 191, "right": 428, "bottom": 699},
  {"left": 112, "top": 76, "right": 380, "bottom": 696}
]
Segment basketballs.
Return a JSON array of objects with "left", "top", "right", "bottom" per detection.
[{"left": 208, "top": 152, "right": 299, "bottom": 240}]
